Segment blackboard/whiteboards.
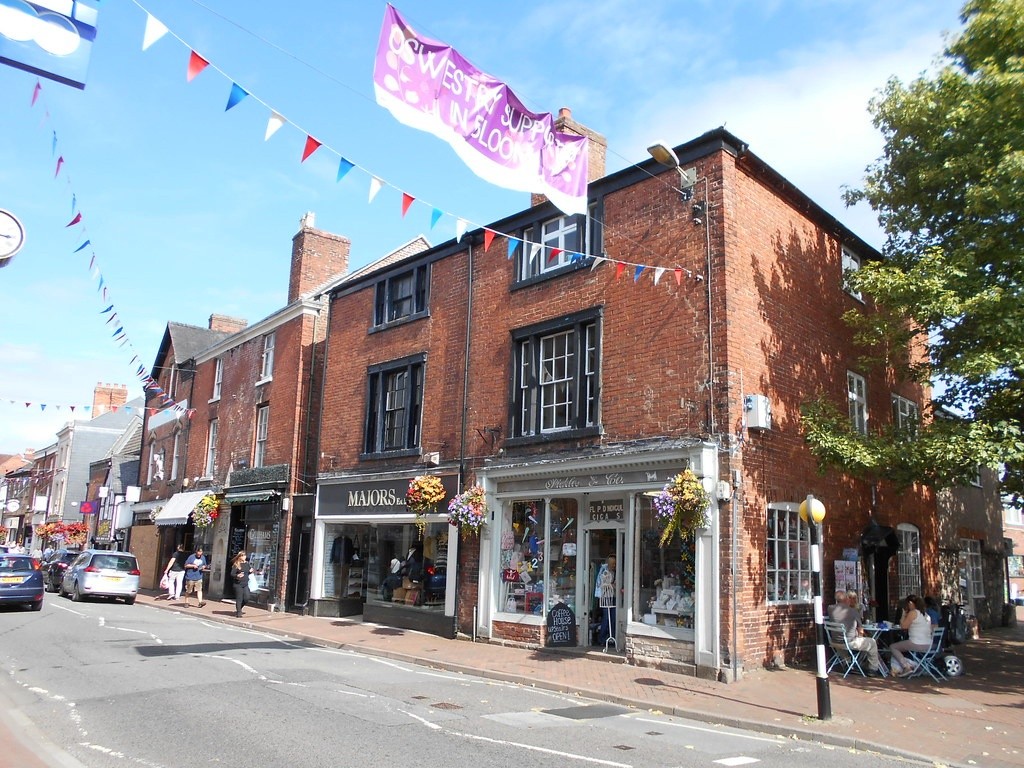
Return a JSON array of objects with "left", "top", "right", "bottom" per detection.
[{"left": 546, "top": 601, "right": 577, "bottom": 648}]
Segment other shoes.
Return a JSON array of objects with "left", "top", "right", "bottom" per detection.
[
  {"left": 237, "top": 611, "right": 245, "bottom": 618},
  {"left": 174, "top": 597, "right": 179, "bottom": 600},
  {"left": 897, "top": 667, "right": 913, "bottom": 677},
  {"left": 184, "top": 603, "right": 189, "bottom": 608},
  {"left": 197, "top": 601, "right": 206, "bottom": 608},
  {"left": 853, "top": 667, "right": 864, "bottom": 674},
  {"left": 167, "top": 594, "right": 174, "bottom": 600},
  {"left": 867, "top": 669, "right": 878, "bottom": 677}
]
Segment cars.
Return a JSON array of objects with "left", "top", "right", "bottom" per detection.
[
  {"left": 0, "top": 553, "right": 44, "bottom": 611},
  {"left": 381, "top": 564, "right": 446, "bottom": 602}
]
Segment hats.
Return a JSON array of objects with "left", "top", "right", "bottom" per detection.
[{"left": 407, "top": 548, "right": 416, "bottom": 561}]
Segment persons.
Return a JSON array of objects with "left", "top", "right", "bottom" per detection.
[
  {"left": 891, "top": 594, "right": 939, "bottom": 677},
  {"left": 827, "top": 590, "right": 879, "bottom": 678},
  {"left": 391, "top": 553, "right": 406, "bottom": 574},
  {"left": 18, "top": 544, "right": 25, "bottom": 552},
  {"left": 184, "top": 545, "right": 207, "bottom": 608},
  {"left": 595, "top": 555, "right": 616, "bottom": 648},
  {"left": 231, "top": 551, "right": 262, "bottom": 617},
  {"left": 45, "top": 545, "right": 54, "bottom": 558},
  {"left": 435, "top": 530, "right": 448, "bottom": 568},
  {"left": 406, "top": 548, "right": 422, "bottom": 583},
  {"left": 165, "top": 544, "right": 187, "bottom": 600},
  {"left": 33, "top": 547, "right": 42, "bottom": 558}
]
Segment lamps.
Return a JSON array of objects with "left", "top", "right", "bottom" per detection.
[
  {"left": 647, "top": 140, "right": 697, "bottom": 187},
  {"left": 423, "top": 451, "right": 439, "bottom": 466}
]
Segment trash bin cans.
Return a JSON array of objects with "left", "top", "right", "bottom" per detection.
[
  {"left": 1003, "top": 603, "right": 1017, "bottom": 627},
  {"left": 964, "top": 614, "right": 980, "bottom": 640}
]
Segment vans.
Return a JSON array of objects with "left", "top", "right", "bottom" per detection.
[{"left": 59, "top": 549, "right": 140, "bottom": 605}]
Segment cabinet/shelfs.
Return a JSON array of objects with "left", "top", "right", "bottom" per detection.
[
  {"left": 348, "top": 569, "right": 364, "bottom": 597},
  {"left": 505, "top": 582, "right": 528, "bottom": 613}
]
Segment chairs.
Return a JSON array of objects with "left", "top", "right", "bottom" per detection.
[
  {"left": 823, "top": 616, "right": 867, "bottom": 679},
  {"left": 907, "top": 627, "right": 949, "bottom": 684}
]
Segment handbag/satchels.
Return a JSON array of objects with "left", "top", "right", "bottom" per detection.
[
  {"left": 248, "top": 573, "right": 258, "bottom": 592},
  {"left": 160, "top": 573, "right": 169, "bottom": 589},
  {"left": 891, "top": 655, "right": 904, "bottom": 674},
  {"left": 349, "top": 534, "right": 365, "bottom": 567}
]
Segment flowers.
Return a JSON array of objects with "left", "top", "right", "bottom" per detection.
[
  {"left": 194, "top": 495, "right": 220, "bottom": 530},
  {"left": 652, "top": 469, "right": 711, "bottom": 548},
  {"left": 447, "top": 484, "right": 491, "bottom": 542},
  {"left": 406, "top": 475, "right": 446, "bottom": 542},
  {"left": 0, "top": 526, "right": 9, "bottom": 542},
  {"left": 148, "top": 504, "right": 165, "bottom": 529},
  {"left": 35, "top": 520, "right": 90, "bottom": 548}
]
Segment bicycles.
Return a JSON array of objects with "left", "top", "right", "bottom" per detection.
[{"left": 942, "top": 596, "right": 970, "bottom": 645}]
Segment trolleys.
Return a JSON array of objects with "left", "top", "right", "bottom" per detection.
[{"left": 936, "top": 597, "right": 964, "bottom": 678}]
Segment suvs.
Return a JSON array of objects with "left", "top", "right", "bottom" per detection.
[{"left": 39, "top": 550, "right": 80, "bottom": 592}]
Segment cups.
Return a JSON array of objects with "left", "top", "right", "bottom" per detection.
[
  {"left": 886, "top": 622, "right": 893, "bottom": 628},
  {"left": 883, "top": 621, "right": 889, "bottom": 626},
  {"left": 866, "top": 619, "right": 869, "bottom": 625}
]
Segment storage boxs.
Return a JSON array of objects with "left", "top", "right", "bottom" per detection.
[{"left": 644, "top": 614, "right": 657, "bottom": 625}]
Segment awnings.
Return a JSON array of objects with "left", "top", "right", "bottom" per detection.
[{"left": 155, "top": 490, "right": 211, "bottom": 525}]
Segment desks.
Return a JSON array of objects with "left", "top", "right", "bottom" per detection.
[
  {"left": 862, "top": 627, "right": 893, "bottom": 677},
  {"left": 651, "top": 609, "right": 694, "bottom": 630}
]
